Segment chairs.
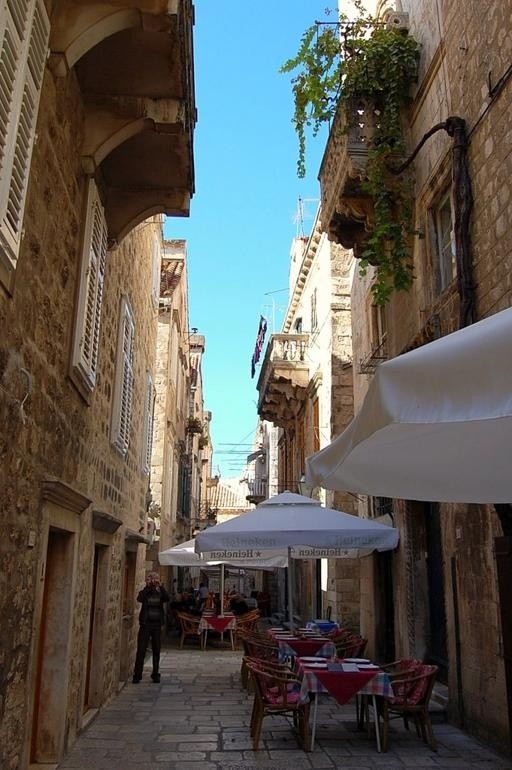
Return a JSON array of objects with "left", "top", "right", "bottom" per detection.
[
  {"left": 235, "top": 622, "right": 442, "bottom": 753},
  {"left": 174, "top": 591, "right": 262, "bottom": 648}
]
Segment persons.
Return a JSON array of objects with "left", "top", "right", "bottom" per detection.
[
  {"left": 171, "top": 567, "right": 270, "bottom": 616},
  {"left": 132, "top": 571, "right": 171, "bottom": 683}
]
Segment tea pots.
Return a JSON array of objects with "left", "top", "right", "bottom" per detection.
[{"left": 323, "top": 642, "right": 337, "bottom": 665}]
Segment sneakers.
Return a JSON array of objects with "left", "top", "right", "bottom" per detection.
[
  {"left": 151, "top": 673, "right": 161, "bottom": 683},
  {"left": 131, "top": 673, "right": 143, "bottom": 684}
]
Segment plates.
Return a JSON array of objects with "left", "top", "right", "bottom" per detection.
[
  {"left": 300, "top": 656, "right": 379, "bottom": 671},
  {"left": 270, "top": 628, "right": 332, "bottom": 642}
]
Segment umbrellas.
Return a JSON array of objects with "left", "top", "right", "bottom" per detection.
[
  {"left": 305, "top": 303, "right": 512, "bottom": 503},
  {"left": 159, "top": 534, "right": 277, "bottom": 616},
  {"left": 192, "top": 488, "right": 399, "bottom": 632}
]
